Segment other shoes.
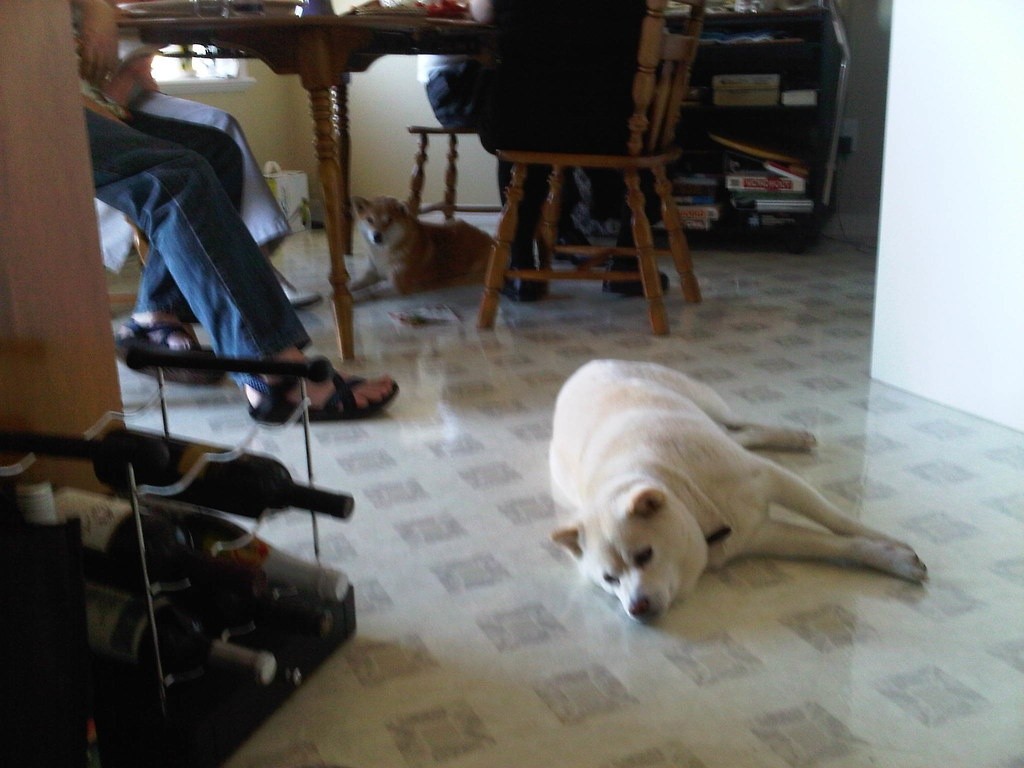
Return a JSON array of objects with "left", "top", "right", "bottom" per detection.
[
  {"left": 500, "top": 268, "right": 543, "bottom": 302},
  {"left": 602, "top": 261, "right": 670, "bottom": 295}
]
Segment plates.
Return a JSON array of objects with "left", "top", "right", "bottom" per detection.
[{"left": 117, "top": 1, "right": 195, "bottom": 17}]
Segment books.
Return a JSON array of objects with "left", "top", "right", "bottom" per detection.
[{"left": 712, "top": 72, "right": 781, "bottom": 105}]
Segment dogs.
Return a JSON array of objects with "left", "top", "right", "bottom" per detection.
[
  {"left": 349, "top": 197, "right": 496, "bottom": 299},
  {"left": 549, "top": 359, "right": 927, "bottom": 623}
]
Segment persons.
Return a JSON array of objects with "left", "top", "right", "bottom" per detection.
[
  {"left": 94, "top": 43, "right": 323, "bottom": 350},
  {"left": 420, "top": 0, "right": 669, "bottom": 300},
  {"left": 70, "top": 0, "right": 400, "bottom": 422}
]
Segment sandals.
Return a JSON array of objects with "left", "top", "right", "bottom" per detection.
[
  {"left": 113, "top": 319, "right": 227, "bottom": 386},
  {"left": 242, "top": 371, "right": 399, "bottom": 423}
]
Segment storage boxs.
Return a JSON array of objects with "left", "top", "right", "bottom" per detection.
[
  {"left": 710, "top": 73, "right": 780, "bottom": 107},
  {"left": 781, "top": 89, "right": 818, "bottom": 105},
  {"left": 262, "top": 159, "right": 309, "bottom": 232}
]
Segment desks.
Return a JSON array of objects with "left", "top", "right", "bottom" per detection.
[{"left": 113, "top": 13, "right": 498, "bottom": 366}]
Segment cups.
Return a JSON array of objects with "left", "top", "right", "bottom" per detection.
[
  {"left": 196, "top": 0, "right": 230, "bottom": 19},
  {"left": 229, "top": 0, "right": 267, "bottom": 17}
]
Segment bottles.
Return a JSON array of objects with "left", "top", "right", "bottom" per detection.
[
  {"left": 53, "top": 484, "right": 349, "bottom": 604},
  {"left": 93, "top": 427, "right": 354, "bottom": 519},
  {"left": 81, "top": 581, "right": 278, "bottom": 687},
  {"left": 15, "top": 479, "right": 59, "bottom": 529}
]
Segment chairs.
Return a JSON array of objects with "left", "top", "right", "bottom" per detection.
[
  {"left": 476, "top": 0, "right": 707, "bottom": 334},
  {"left": 408, "top": 124, "right": 479, "bottom": 223}
]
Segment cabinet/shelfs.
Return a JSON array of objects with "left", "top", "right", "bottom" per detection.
[{"left": 651, "top": 0, "right": 853, "bottom": 252}]
